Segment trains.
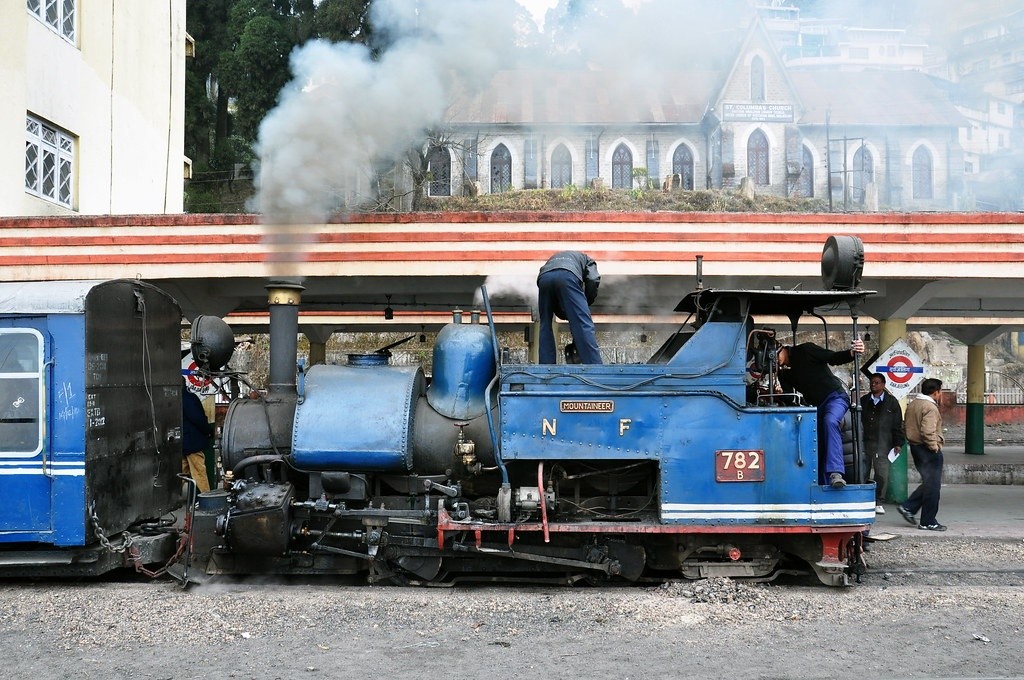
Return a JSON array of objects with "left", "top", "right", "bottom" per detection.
[{"left": 0, "top": 236, "right": 877, "bottom": 589}]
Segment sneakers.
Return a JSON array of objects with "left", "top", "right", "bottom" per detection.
[
  {"left": 918, "top": 523, "right": 947, "bottom": 531},
  {"left": 830, "top": 473, "right": 846, "bottom": 487},
  {"left": 898, "top": 505, "right": 916, "bottom": 525}
]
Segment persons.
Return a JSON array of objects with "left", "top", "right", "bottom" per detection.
[
  {"left": 859, "top": 374, "right": 905, "bottom": 515},
  {"left": 776, "top": 336, "right": 866, "bottom": 488},
  {"left": 537, "top": 250, "right": 603, "bottom": 364},
  {"left": 182, "top": 376, "right": 212, "bottom": 493},
  {"left": 897, "top": 379, "right": 947, "bottom": 531},
  {"left": 242, "top": 390, "right": 262, "bottom": 400}
]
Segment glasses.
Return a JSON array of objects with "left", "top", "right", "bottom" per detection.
[{"left": 869, "top": 381, "right": 882, "bottom": 385}]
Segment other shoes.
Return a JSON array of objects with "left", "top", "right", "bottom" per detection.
[{"left": 876, "top": 505, "right": 885, "bottom": 514}]
